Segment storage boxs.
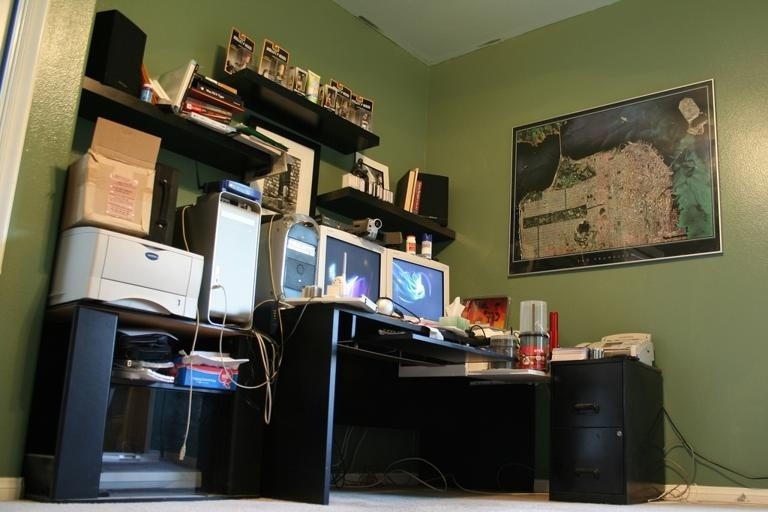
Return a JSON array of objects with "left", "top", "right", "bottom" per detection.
[{"left": 59, "top": 115, "right": 166, "bottom": 238}]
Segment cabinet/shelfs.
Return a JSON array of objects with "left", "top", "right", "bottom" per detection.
[
  {"left": 548, "top": 356, "right": 666, "bottom": 504},
  {"left": 26, "top": 297, "right": 253, "bottom": 502},
  {"left": 83, "top": 65, "right": 455, "bottom": 259}
]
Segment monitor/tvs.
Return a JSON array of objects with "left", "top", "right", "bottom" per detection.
[
  {"left": 314, "top": 225, "right": 387, "bottom": 303},
  {"left": 387, "top": 249, "right": 449, "bottom": 323}
]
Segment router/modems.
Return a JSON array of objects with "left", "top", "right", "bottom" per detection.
[{"left": 195, "top": 159, "right": 261, "bottom": 201}]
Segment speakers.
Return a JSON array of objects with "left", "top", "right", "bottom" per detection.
[
  {"left": 197, "top": 336, "right": 271, "bottom": 495},
  {"left": 417, "top": 173, "right": 449, "bottom": 227},
  {"left": 20, "top": 306, "right": 120, "bottom": 502}
]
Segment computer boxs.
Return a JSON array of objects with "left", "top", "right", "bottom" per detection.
[
  {"left": 257, "top": 213, "right": 320, "bottom": 298},
  {"left": 175, "top": 191, "right": 262, "bottom": 334}
]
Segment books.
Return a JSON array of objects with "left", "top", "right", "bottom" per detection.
[
  {"left": 238, "top": 121, "right": 291, "bottom": 158},
  {"left": 394, "top": 167, "right": 422, "bottom": 216},
  {"left": 178, "top": 64, "right": 245, "bottom": 138},
  {"left": 110, "top": 329, "right": 178, "bottom": 383}
]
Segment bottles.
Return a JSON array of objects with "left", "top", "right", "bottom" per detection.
[
  {"left": 406, "top": 235, "right": 416, "bottom": 255},
  {"left": 421, "top": 232, "right": 433, "bottom": 259},
  {"left": 140, "top": 84, "right": 153, "bottom": 104}
]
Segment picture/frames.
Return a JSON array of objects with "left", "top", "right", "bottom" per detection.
[
  {"left": 243, "top": 114, "right": 321, "bottom": 225},
  {"left": 507, "top": 79, "right": 722, "bottom": 277}
]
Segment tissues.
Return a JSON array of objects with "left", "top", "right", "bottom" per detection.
[{"left": 439, "top": 296, "right": 470, "bottom": 331}]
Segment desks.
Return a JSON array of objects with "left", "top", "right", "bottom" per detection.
[{"left": 245, "top": 300, "right": 541, "bottom": 504}]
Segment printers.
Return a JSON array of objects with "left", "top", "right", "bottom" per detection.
[{"left": 48, "top": 227, "right": 205, "bottom": 318}]
